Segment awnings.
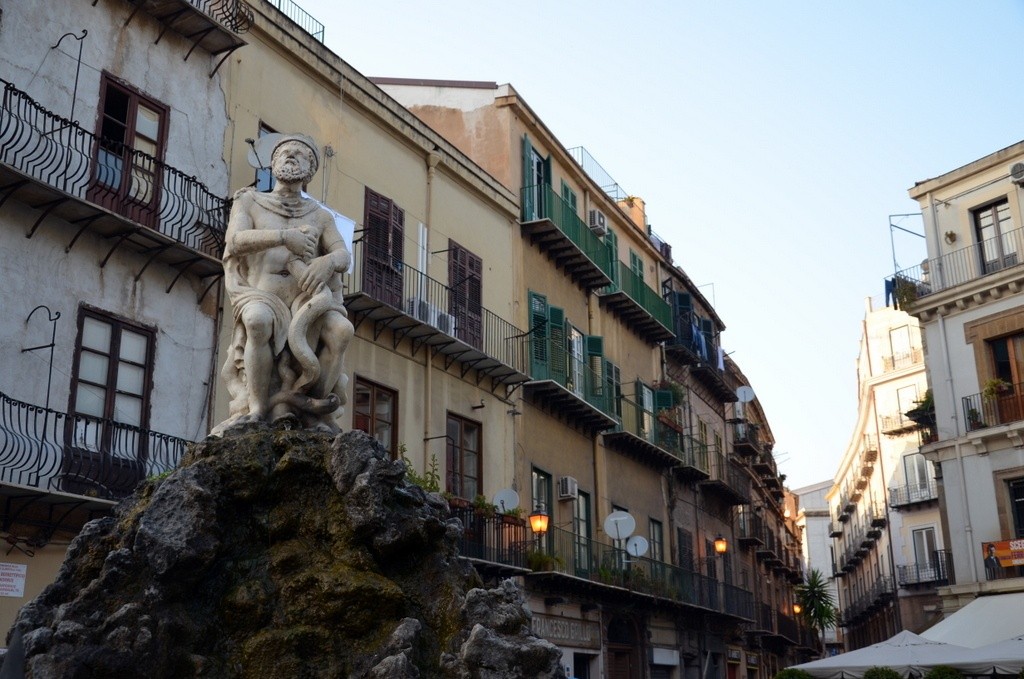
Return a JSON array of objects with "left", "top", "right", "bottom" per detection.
[{"left": 920, "top": 593, "right": 1024, "bottom": 647}]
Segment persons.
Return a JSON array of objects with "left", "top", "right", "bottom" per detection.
[
  {"left": 984, "top": 544, "right": 1003, "bottom": 578},
  {"left": 223, "top": 134, "right": 354, "bottom": 423}
]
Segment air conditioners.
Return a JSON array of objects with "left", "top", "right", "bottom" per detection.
[
  {"left": 589, "top": 208, "right": 607, "bottom": 235},
  {"left": 1007, "top": 157, "right": 1024, "bottom": 185},
  {"left": 408, "top": 299, "right": 459, "bottom": 337},
  {"left": 561, "top": 474, "right": 579, "bottom": 498}
]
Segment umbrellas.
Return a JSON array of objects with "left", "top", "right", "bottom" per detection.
[{"left": 785, "top": 629, "right": 1023, "bottom": 679}]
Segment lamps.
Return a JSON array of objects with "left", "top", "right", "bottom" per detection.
[
  {"left": 528, "top": 505, "right": 550, "bottom": 537},
  {"left": 714, "top": 533, "right": 727, "bottom": 557},
  {"left": 793, "top": 600, "right": 801, "bottom": 616}
]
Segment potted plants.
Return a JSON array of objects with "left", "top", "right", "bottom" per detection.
[
  {"left": 589, "top": 564, "right": 680, "bottom": 603},
  {"left": 450, "top": 493, "right": 525, "bottom": 525},
  {"left": 529, "top": 548, "right": 565, "bottom": 572},
  {"left": 920, "top": 380, "right": 1011, "bottom": 442},
  {"left": 659, "top": 407, "right": 684, "bottom": 433}
]
